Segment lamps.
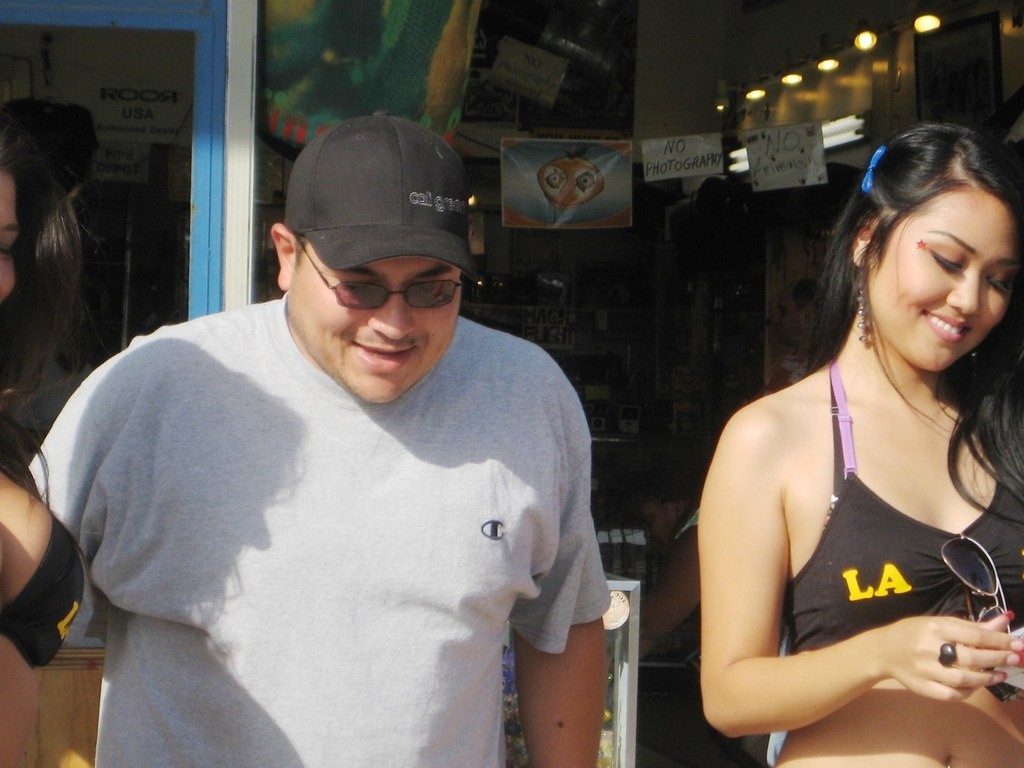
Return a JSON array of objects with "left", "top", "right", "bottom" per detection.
[
  {"left": 714, "top": 78, "right": 739, "bottom": 110},
  {"left": 817, "top": 34, "right": 840, "bottom": 71},
  {"left": 780, "top": 48, "right": 803, "bottom": 85},
  {"left": 745, "top": 67, "right": 767, "bottom": 100},
  {"left": 852, "top": 19, "right": 878, "bottom": 52}
]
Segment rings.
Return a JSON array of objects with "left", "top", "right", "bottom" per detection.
[{"left": 939, "top": 643, "right": 957, "bottom": 666}]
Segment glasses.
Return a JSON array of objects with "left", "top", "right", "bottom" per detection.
[
  {"left": 294, "top": 237, "right": 467, "bottom": 308},
  {"left": 937, "top": 534, "right": 1024, "bottom": 704}
]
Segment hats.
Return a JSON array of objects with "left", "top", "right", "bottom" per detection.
[
  {"left": 278, "top": 115, "right": 487, "bottom": 290},
  {"left": 0, "top": 100, "right": 99, "bottom": 203}
]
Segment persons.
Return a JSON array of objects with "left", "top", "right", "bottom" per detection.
[
  {"left": 1, "top": 105, "right": 85, "bottom": 669},
  {"left": 24, "top": 113, "right": 612, "bottom": 768},
  {"left": 698, "top": 122, "right": 1023, "bottom": 768}
]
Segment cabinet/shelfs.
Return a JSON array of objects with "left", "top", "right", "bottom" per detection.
[{"left": 459, "top": 268, "right": 710, "bottom": 669}]
